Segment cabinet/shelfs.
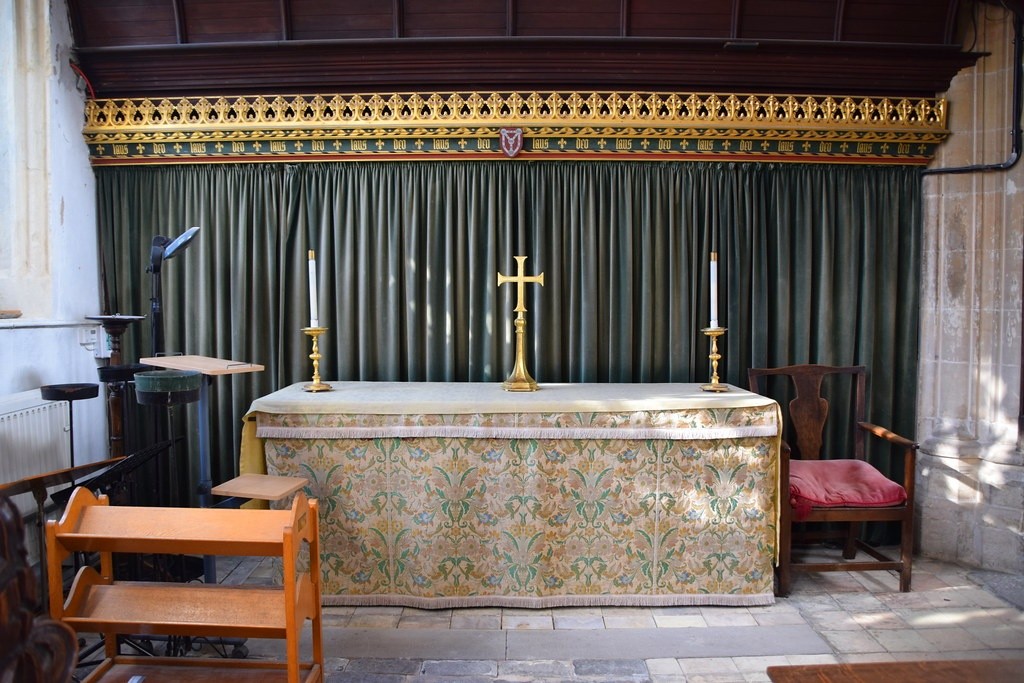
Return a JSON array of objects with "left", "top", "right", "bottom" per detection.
[{"left": 46, "top": 488, "right": 324, "bottom": 683}]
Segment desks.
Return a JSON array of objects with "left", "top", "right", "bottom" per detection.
[{"left": 234, "top": 383, "right": 784, "bottom": 608}]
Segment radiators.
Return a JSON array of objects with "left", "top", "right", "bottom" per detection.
[{"left": 0, "top": 388, "right": 69, "bottom": 486}]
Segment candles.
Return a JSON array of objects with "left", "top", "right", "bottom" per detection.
[
  {"left": 310, "top": 249, "right": 318, "bottom": 327},
  {"left": 710, "top": 251, "right": 718, "bottom": 328}
]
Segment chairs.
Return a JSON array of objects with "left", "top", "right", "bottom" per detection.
[{"left": 750, "top": 365, "right": 919, "bottom": 599}]
